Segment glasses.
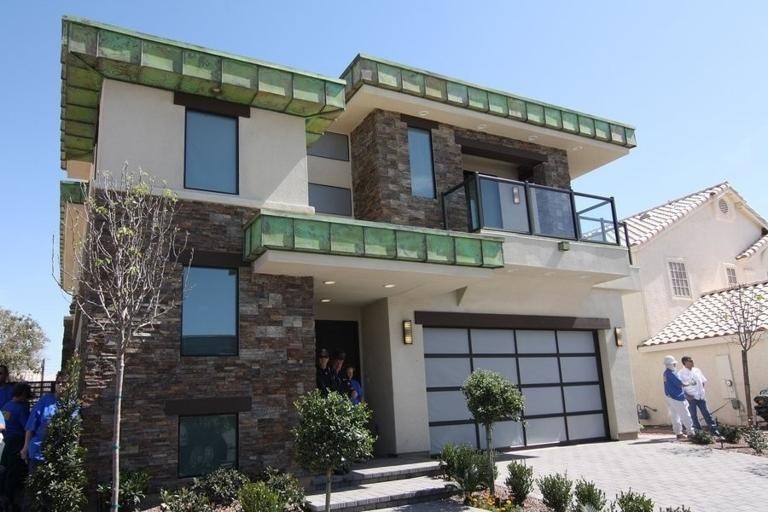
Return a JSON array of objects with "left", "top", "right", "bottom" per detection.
[
  {"left": 558, "top": 241, "right": 569, "bottom": 251},
  {"left": 402, "top": 319, "right": 413, "bottom": 345},
  {"left": 614, "top": 326, "right": 623, "bottom": 346}
]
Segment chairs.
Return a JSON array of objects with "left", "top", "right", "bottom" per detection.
[{"left": 676, "top": 434, "right": 687, "bottom": 439}]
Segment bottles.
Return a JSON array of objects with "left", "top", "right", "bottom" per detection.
[
  {"left": 335, "top": 349, "right": 346, "bottom": 359},
  {"left": 664, "top": 355, "right": 677, "bottom": 370},
  {"left": 319, "top": 348, "right": 329, "bottom": 358}
]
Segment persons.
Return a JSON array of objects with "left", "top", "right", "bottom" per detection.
[
  {"left": 0, "top": 362, "right": 80, "bottom": 512},
  {"left": 316, "top": 346, "right": 339, "bottom": 398},
  {"left": 329, "top": 349, "right": 359, "bottom": 404},
  {"left": 662, "top": 355, "right": 699, "bottom": 439},
  {"left": 343, "top": 364, "right": 365, "bottom": 407},
  {"left": 676, "top": 356, "right": 727, "bottom": 441}
]
